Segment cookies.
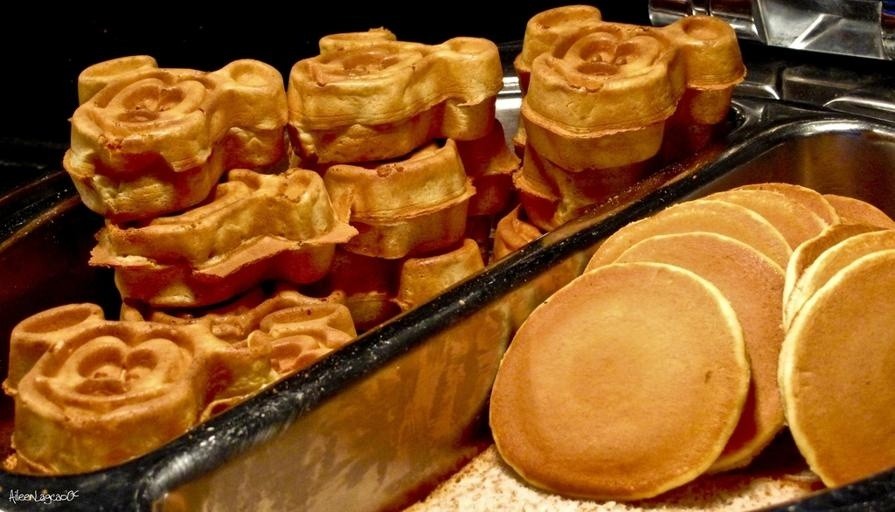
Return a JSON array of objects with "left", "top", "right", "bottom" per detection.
[{"left": 494, "top": 181, "right": 895, "bottom": 501}]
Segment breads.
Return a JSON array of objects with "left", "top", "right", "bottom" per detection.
[
  {"left": 399, "top": 445, "right": 817, "bottom": 510},
  {"left": 3, "top": 5, "right": 750, "bottom": 477}
]
